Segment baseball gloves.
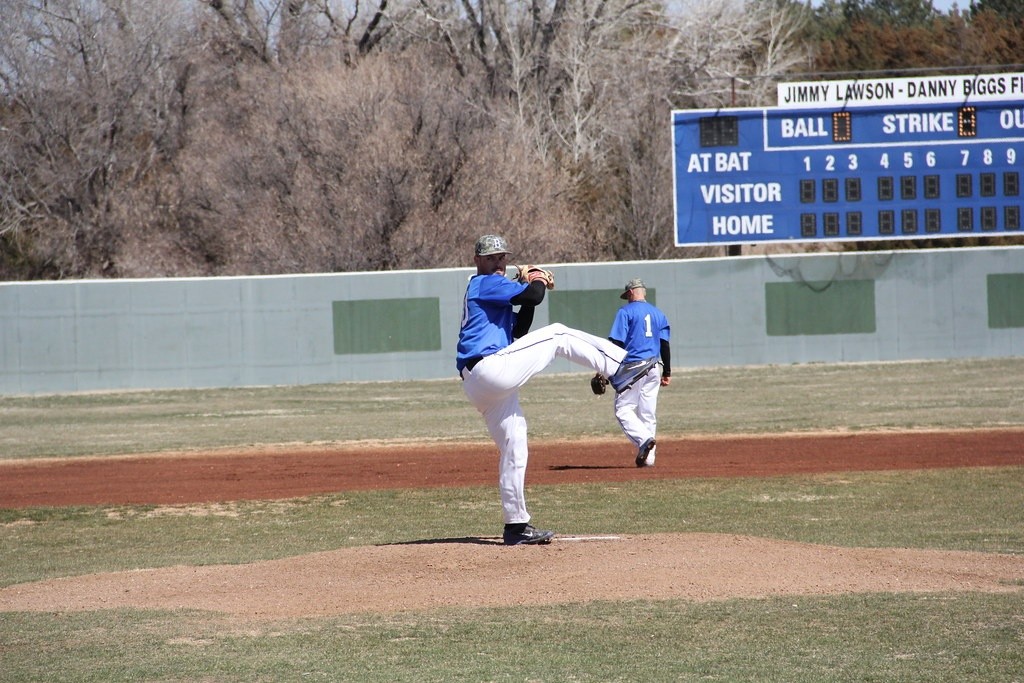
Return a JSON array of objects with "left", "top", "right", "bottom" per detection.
[
  {"left": 591, "top": 372, "right": 610, "bottom": 395},
  {"left": 514, "top": 263, "right": 555, "bottom": 291}
]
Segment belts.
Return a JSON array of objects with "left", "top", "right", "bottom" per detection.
[{"left": 460, "top": 355, "right": 482, "bottom": 381}]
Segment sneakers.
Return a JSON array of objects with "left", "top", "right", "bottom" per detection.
[
  {"left": 502, "top": 524, "right": 554, "bottom": 545},
  {"left": 636, "top": 437, "right": 657, "bottom": 466},
  {"left": 609, "top": 357, "right": 656, "bottom": 393}
]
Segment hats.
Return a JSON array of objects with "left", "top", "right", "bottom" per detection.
[
  {"left": 621, "top": 279, "right": 645, "bottom": 300},
  {"left": 475, "top": 235, "right": 512, "bottom": 257}
]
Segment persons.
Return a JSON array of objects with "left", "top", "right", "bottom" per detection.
[
  {"left": 590, "top": 279, "right": 671, "bottom": 466},
  {"left": 457, "top": 234, "right": 657, "bottom": 545}
]
